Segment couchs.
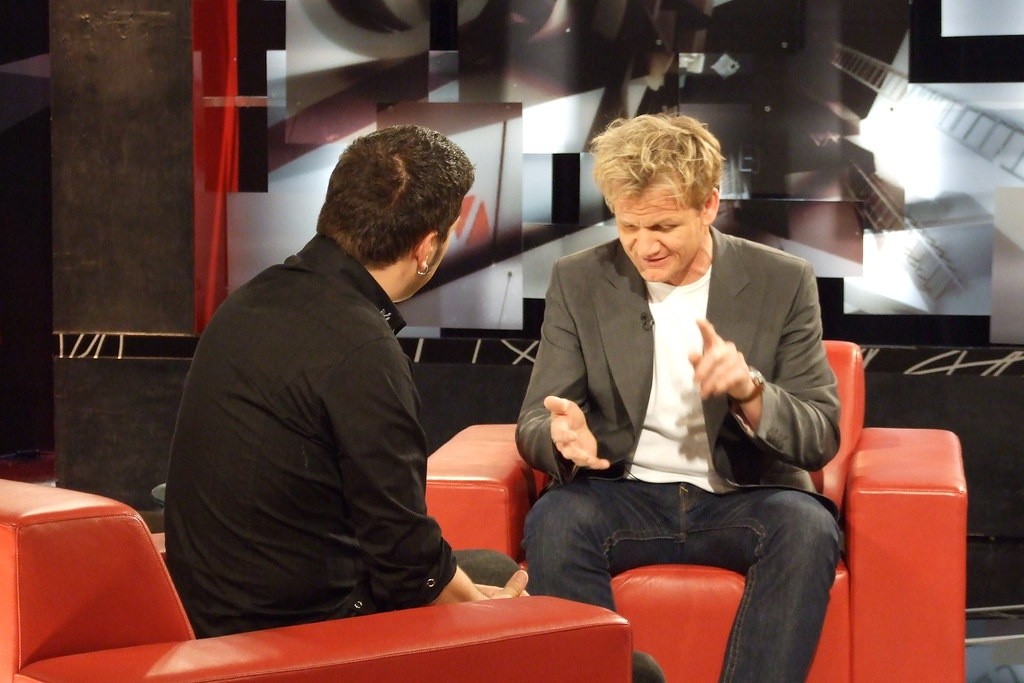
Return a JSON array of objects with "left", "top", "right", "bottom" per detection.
[
  {"left": 0, "top": 480, "right": 634, "bottom": 683},
  {"left": 425, "top": 339, "right": 967, "bottom": 683}
]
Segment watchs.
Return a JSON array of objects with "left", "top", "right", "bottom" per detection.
[{"left": 728, "top": 365, "right": 767, "bottom": 403}]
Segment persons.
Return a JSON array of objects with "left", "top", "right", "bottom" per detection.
[
  {"left": 163, "top": 123, "right": 663, "bottom": 683},
  {"left": 514, "top": 113, "right": 840, "bottom": 682}
]
